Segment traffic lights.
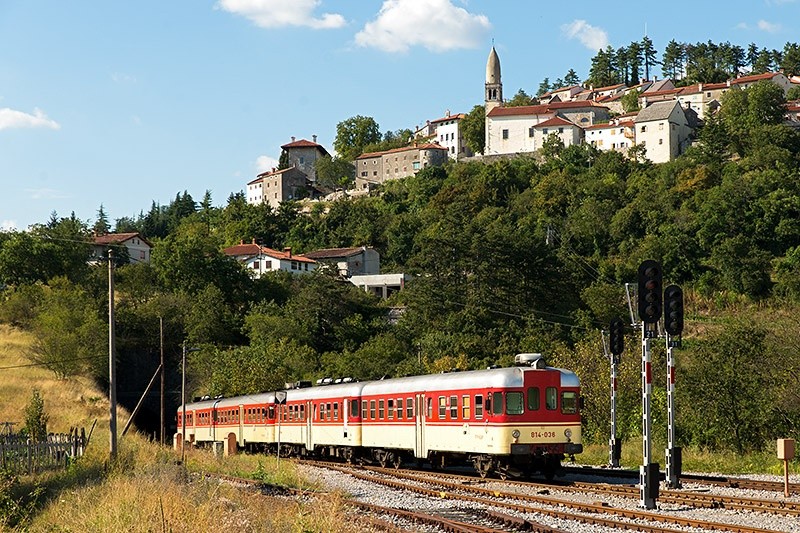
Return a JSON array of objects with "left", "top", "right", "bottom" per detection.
[
  {"left": 610, "top": 317, "right": 624, "bottom": 355},
  {"left": 664, "top": 285, "right": 684, "bottom": 334},
  {"left": 637, "top": 260, "right": 662, "bottom": 323}
]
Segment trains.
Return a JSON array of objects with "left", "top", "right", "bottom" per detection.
[{"left": 177, "top": 353, "right": 585, "bottom": 479}]
